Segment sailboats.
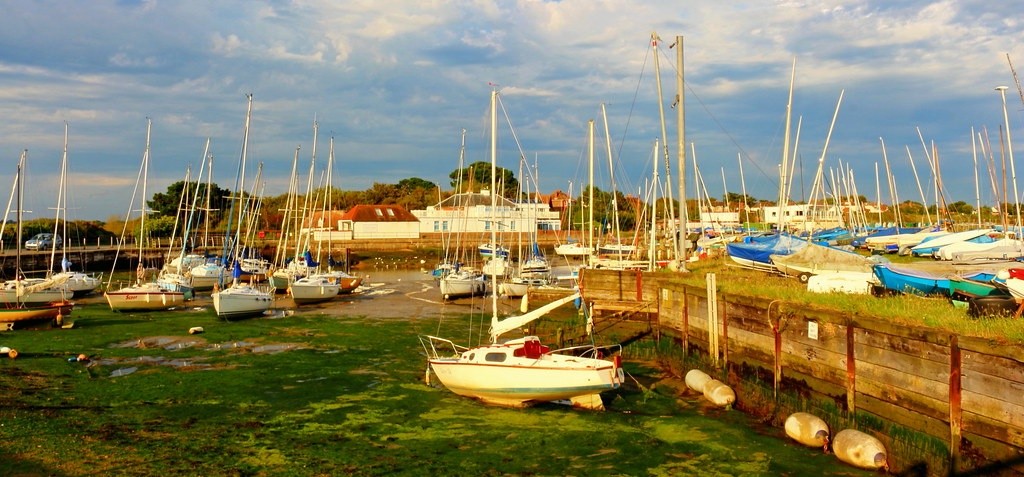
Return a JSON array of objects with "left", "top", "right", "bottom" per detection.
[
  {"left": 432, "top": 34, "right": 1024, "bottom": 315},
  {"left": 0, "top": 93, "right": 366, "bottom": 329},
  {"left": 416, "top": 82, "right": 625, "bottom": 404}
]
